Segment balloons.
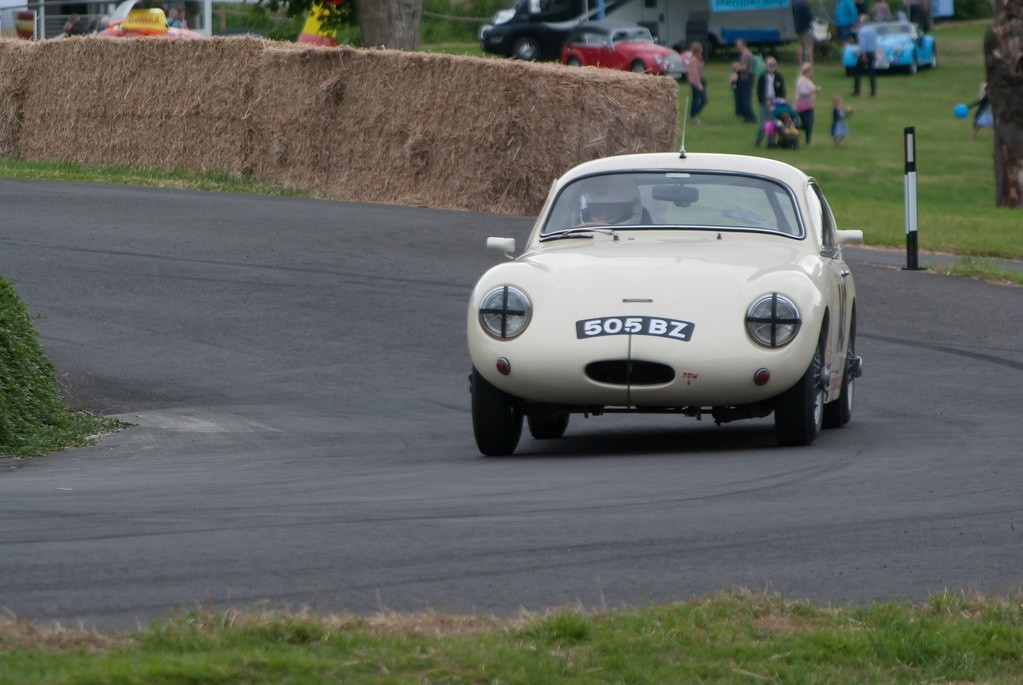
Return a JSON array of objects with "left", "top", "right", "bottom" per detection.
[
  {"left": 954, "top": 103, "right": 969, "bottom": 118},
  {"left": 765, "top": 121, "right": 775, "bottom": 134}
]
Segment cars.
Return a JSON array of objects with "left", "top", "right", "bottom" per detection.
[
  {"left": 0, "top": 0, "right": 213, "bottom": 39},
  {"left": 465, "top": 151, "right": 866, "bottom": 458},
  {"left": 559, "top": 19, "right": 688, "bottom": 83},
  {"left": 474, "top": 0, "right": 598, "bottom": 64},
  {"left": 841, "top": 19, "right": 938, "bottom": 76}
]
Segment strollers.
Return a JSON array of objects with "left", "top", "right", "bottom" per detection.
[{"left": 769, "top": 101, "right": 801, "bottom": 149}]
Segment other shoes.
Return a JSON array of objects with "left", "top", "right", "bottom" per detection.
[{"left": 693, "top": 116, "right": 701, "bottom": 124}]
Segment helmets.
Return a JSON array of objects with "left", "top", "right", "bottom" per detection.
[{"left": 582, "top": 183, "right": 644, "bottom": 226}]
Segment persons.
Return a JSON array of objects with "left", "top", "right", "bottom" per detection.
[
  {"left": 688, "top": 42, "right": 709, "bottom": 125},
  {"left": 783, "top": 112, "right": 799, "bottom": 134},
  {"left": 574, "top": 175, "right": 654, "bottom": 226},
  {"left": 729, "top": 39, "right": 757, "bottom": 124},
  {"left": 968, "top": 85, "right": 995, "bottom": 144},
  {"left": 63, "top": 13, "right": 110, "bottom": 36},
  {"left": 792, "top": 0, "right": 815, "bottom": 66},
  {"left": 161, "top": 3, "right": 189, "bottom": 29},
  {"left": 869, "top": 0, "right": 933, "bottom": 32},
  {"left": 834, "top": 0, "right": 858, "bottom": 40},
  {"left": 794, "top": 62, "right": 822, "bottom": 144},
  {"left": 830, "top": 95, "right": 853, "bottom": 146},
  {"left": 851, "top": 13, "right": 877, "bottom": 97},
  {"left": 753, "top": 56, "right": 786, "bottom": 147}
]
